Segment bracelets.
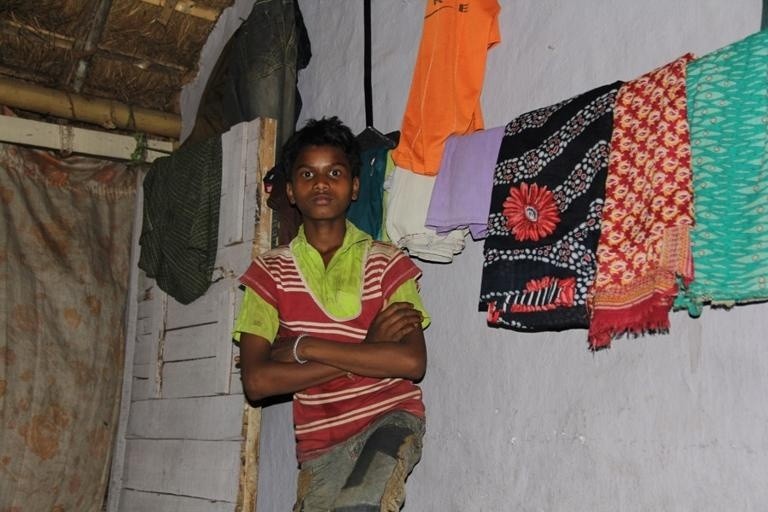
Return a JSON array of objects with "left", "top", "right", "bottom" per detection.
[{"left": 292, "top": 334, "right": 312, "bottom": 366}]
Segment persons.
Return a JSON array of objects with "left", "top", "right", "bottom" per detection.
[{"left": 231, "top": 116, "right": 427, "bottom": 512}]
